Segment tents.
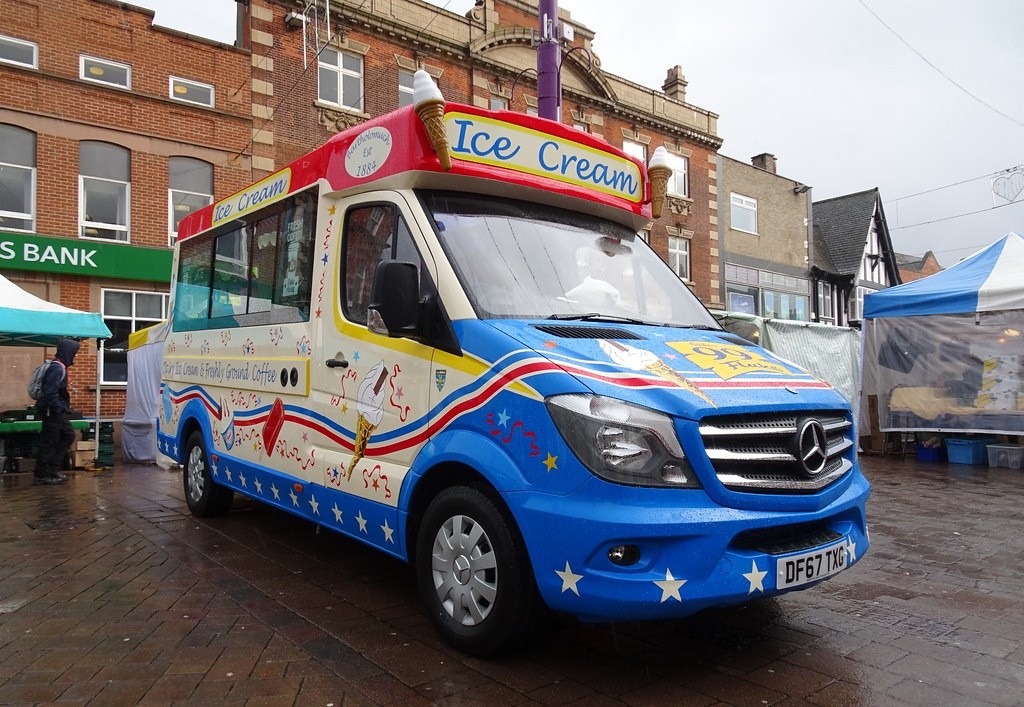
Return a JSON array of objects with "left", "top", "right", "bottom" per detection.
[
  {"left": 707, "top": 309, "right": 861, "bottom": 461},
  {"left": 0, "top": 273, "right": 113, "bottom": 469},
  {"left": 860, "top": 231, "right": 1024, "bottom": 436}
]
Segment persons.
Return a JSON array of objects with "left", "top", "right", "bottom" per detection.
[{"left": 32, "top": 340, "right": 80, "bottom": 485}]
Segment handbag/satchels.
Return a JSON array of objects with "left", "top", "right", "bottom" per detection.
[
  {"left": 878, "top": 339, "right": 919, "bottom": 375},
  {"left": 916, "top": 329, "right": 937, "bottom": 353},
  {"left": 940, "top": 335, "right": 969, "bottom": 361}
]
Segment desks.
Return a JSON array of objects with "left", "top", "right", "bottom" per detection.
[{"left": 0, "top": 421, "right": 90, "bottom": 469}]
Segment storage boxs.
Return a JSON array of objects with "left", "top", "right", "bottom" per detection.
[
  {"left": 912, "top": 446, "right": 947, "bottom": 463},
  {"left": 943, "top": 438, "right": 995, "bottom": 465},
  {"left": 985, "top": 443, "right": 1024, "bottom": 470},
  {"left": 80, "top": 422, "right": 116, "bottom": 466},
  {"left": 71, "top": 441, "right": 96, "bottom": 467}
]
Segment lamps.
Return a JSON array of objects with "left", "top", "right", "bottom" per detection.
[
  {"left": 794, "top": 184, "right": 813, "bottom": 193},
  {"left": 285, "top": 11, "right": 311, "bottom": 29}
]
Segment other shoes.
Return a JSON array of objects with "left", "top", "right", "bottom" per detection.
[
  {"left": 31, "top": 475, "right": 63, "bottom": 485},
  {"left": 51, "top": 471, "right": 69, "bottom": 481}
]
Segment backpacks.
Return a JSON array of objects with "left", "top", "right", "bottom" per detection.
[{"left": 27, "top": 358, "right": 66, "bottom": 400}]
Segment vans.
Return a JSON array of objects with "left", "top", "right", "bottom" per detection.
[{"left": 153, "top": 70, "right": 873, "bottom": 653}]
